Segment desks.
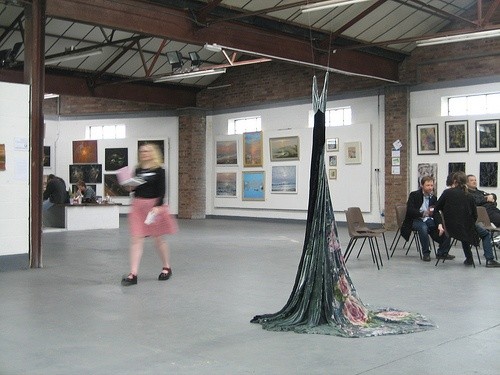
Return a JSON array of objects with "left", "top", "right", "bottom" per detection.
[{"left": 44, "top": 202, "right": 122, "bottom": 232}]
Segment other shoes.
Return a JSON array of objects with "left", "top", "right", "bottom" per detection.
[
  {"left": 423, "top": 255, "right": 430, "bottom": 260},
  {"left": 465, "top": 258, "right": 472, "bottom": 265},
  {"left": 486, "top": 259, "right": 500, "bottom": 267},
  {"left": 158, "top": 267, "right": 171, "bottom": 280},
  {"left": 436, "top": 253, "right": 455, "bottom": 260},
  {"left": 121, "top": 272, "right": 137, "bottom": 285}
]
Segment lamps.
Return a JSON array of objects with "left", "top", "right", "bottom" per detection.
[
  {"left": 298, "top": 0, "right": 366, "bottom": 15},
  {"left": 164, "top": 50, "right": 184, "bottom": 70},
  {"left": 415, "top": 29, "right": 500, "bottom": 47},
  {"left": 188, "top": 51, "right": 203, "bottom": 70},
  {"left": 153, "top": 67, "right": 228, "bottom": 83},
  {"left": 43, "top": 47, "right": 105, "bottom": 64}
]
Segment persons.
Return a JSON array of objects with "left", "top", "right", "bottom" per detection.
[
  {"left": 433, "top": 173, "right": 500, "bottom": 267},
  {"left": 77, "top": 181, "right": 96, "bottom": 201},
  {"left": 42, "top": 174, "right": 66, "bottom": 226},
  {"left": 400, "top": 176, "right": 455, "bottom": 261},
  {"left": 466, "top": 175, "right": 500, "bottom": 249},
  {"left": 121, "top": 143, "right": 172, "bottom": 284}
]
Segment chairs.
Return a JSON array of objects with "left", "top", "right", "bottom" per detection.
[
  {"left": 435, "top": 210, "right": 481, "bottom": 268},
  {"left": 348, "top": 206, "right": 391, "bottom": 263},
  {"left": 476, "top": 206, "right": 500, "bottom": 260},
  {"left": 343, "top": 209, "right": 385, "bottom": 271},
  {"left": 392, "top": 204, "right": 423, "bottom": 260}
]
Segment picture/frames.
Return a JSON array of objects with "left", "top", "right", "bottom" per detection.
[
  {"left": 269, "top": 135, "right": 301, "bottom": 162},
  {"left": 329, "top": 169, "right": 337, "bottom": 179},
  {"left": 241, "top": 130, "right": 264, "bottom": 167},
  {"left": 326, "top": 136, "right": 340, "bottom": 152},
  {"left": 416, "top": 123, "right": 440, "bottom": 156},
  {"left": 329, "top": 155, "right": 337, "bottom": 166},
  {"left": 215, "top": 139, "right": 240, "bottom": 167},
  {"left": 214, "top": 170, "right": 239, "bottom": 199},
  {"left": 270, "top": 164, "right": 298, "bottom": 194},
  {"left": 474, "top": 119, "right": 500, "bottom": 153},
  {"left": 241, "top": 169, "right": 267, "bottom": 202},
  {"left": 444, "top": 119, "right": 469, "bottom": 153},
  {"left": 344, "top": 140, "right": 362, "bottom": 165}
]
